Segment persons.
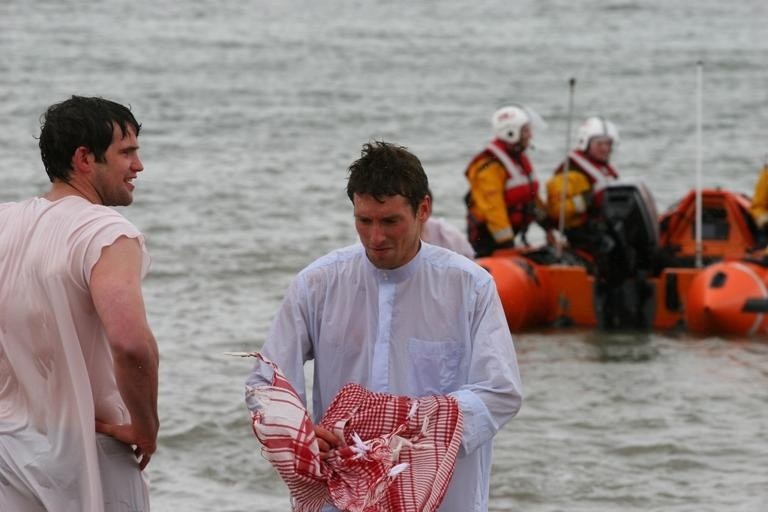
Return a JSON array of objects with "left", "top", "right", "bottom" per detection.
[
  {"left": 464, "top": 100, "right": 555, "bottom": 258},
  {"left": 0, "top": 93, "right": 161, "bottom": 511},
  {"left": 245, "top": 141, "right": 524, "bottom": 509},
  {"left": 748, "top": 163, "right": 768, "bottom": 249},
  {"left": 419, "top": 216, "right": 477, "bottom": 263},
  {"left": 546, "top": 115, "right": 620, "bottom": 250}
]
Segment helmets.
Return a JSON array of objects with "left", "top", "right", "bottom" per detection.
[
  {"left": 492, "top": 102, "right": 535, "bottom": 145},
  {"left": 575, "top": 116, "right": 620, "bottom": 155}
]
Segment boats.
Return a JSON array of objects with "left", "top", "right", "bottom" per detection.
[{"left": 470, "top": 188, "right": 768, "bottom": 339}]
